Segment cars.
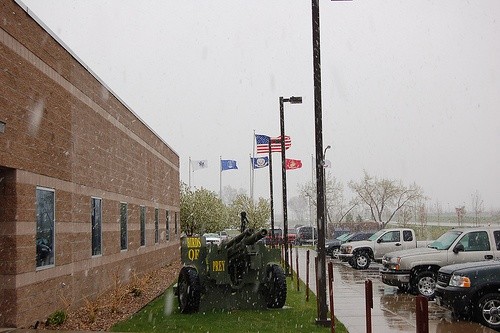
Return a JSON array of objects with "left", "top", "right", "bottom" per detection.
[
  {"left": 314, "top": 230, "right": 376, "bottom": 260},
  {"left": 433, "top": 254, "right": 500, "bottom": 331},
  {"left": 265, "top": 227, "right": 299, "bottom": 247},
  {"left": 200, "top": 233, "right": 222, "bottom": 251}
]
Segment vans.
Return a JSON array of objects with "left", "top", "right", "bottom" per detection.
[
  {"left": 216, "top": 231, "right": 228, "bottom": 242},
  {"left": 294, "top": 225, "right": 318, "bottom": 247}
]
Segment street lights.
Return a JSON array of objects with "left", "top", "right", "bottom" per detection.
[{"left": 278, "top": 95, "right": 303, "bottom": 276}]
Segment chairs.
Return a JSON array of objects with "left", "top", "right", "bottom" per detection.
[{"left": 468, "top": 236, "right": 485, "bottom": 250}]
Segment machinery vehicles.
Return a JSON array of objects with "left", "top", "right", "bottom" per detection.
[{"left": 172, "top": 211, "right": 288, "bottom": 315}]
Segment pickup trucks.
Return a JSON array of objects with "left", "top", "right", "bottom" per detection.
[
  {"left": 338, "top": 228, "right": 437, "bottom": 270},
  {"left": 379, "top": 225, "right": 500, "bottom": 301}
]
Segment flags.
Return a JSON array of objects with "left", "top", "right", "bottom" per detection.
[
  {"left": 256, "top": 135, "right": 292, "bottom": 154},
  {"left": 285, "top": 159, "right": 302, "bottom": 170},
  {"left": 221, "top": 159, "right": 238, "bottom": 171},
  {"left": 191, "top": 159, "right": 208, "bottom": 172},
  {"left": 251, "top": 156, "right": 269, "bottom": 169}
]
